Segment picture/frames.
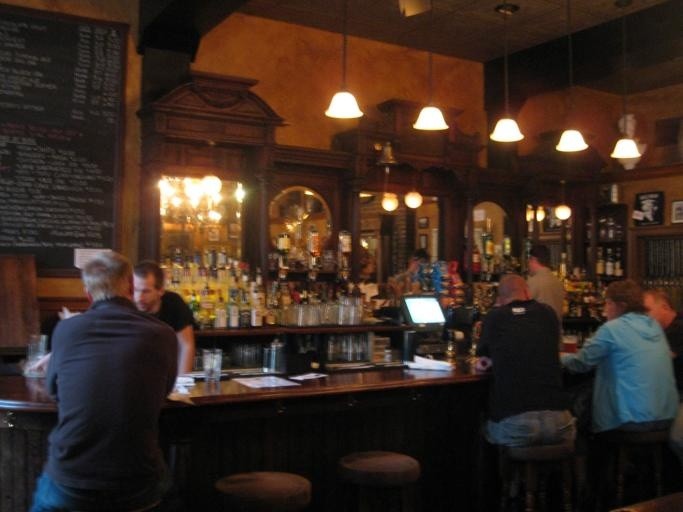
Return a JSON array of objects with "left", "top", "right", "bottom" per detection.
[
  {"left": 416, "top": 215, "right": 429, "bottom": 229},
  {"left": 670, "top": 198, "right": 682, "bottom": 224},
  {"left": 635, "top": 190, "right": 664, "bottom": 225},
  {"left": 418, "top": 233, "right": 428, "bottom": 249}
]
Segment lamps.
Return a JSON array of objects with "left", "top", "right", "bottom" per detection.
[
  {"left": 480, "top": 1, "right": 527, "bottom": 142},
  {"left": 320, "top": 0, "right": 365, "bottom": 122},
  {"left": 408, "top": 1, "right": 451, "bottom": 132},
  {"left": 545, "top": 2, "right": 589, "bottom": 153},
  {"left": 605, "top": 0, "right": 642, "bottom": 161}
]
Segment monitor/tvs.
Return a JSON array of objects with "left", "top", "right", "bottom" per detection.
[{"left": 400, "top": 295, "right": 447, "bottom": 330}]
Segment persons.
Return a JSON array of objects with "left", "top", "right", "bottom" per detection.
[
  {"left": 527, "top": 245, "right": 563, "bottom": 333},
  {"left": 30, "top": 252, "right": 179, "bottom": 512},
  {"left": 557, "top": 281, "right": 679, "bottom": 457},
  {"left": 395, "top": 248, "right": 431, "bottom": 286},
  {"left": 642, "top": 289, "right": 682, "bottom": 460},
  {"left": 132, "top": 260, "right": 197, "bottom": 376},
  {"left": 474, "top": 273, "right": 578, "bottom": 448}
]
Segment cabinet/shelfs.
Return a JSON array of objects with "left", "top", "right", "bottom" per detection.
[{"left": 579, "top": 201, "right": 628, "bottom": 283}]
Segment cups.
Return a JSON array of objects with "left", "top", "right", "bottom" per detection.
[
  {"left": 326, "top": 335, "right": 367, "bottom": 361},
  {"left": 201, "top": 348, "right": 223, "bottom": 382},
  {"left": 286, "top": 295, "right": 364, "bottom": 327},
  {"left": 236, "top": 344, "right": 258, "bottom": 368},
  {"left": 25, "top": 332, "right": 46, "bottom": 373}
]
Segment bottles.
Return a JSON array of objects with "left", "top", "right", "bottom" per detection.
[
  {"left": 563, "top": 284, "right": 606, "bottom": 343},
  {"left": 556, "top": 250, "right": 591, "bottom": 281},
  {"left": 644, "top": 278, "right": 683, "bottom": 294},
  {"left": 445, "top": 303, "right": 483, "bottom": 357},
  {"left": 160, "top": 245, "right": 266, "bottom": 327},
  {"left": 269, "top": 225, "right": 352, "bottom": 325},
  {"left": 262, "top": 339, "right": 286, "bottom": 373},
  {"left": 468, "top": 233, "right": 522, "bottom": 275},
  {"left": 384, "top": 347, "right": 392, "bottom": 362},
  {"left": 596, "top": 216, "right": 624, "bottom": 277}
]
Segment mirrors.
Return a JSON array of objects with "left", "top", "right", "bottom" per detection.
[
  {"left": 265, "top": 186, "right": 335, "bottom": 263},
  {"left": 460, "top": 201, "right": 514, "bottom": 278},
  {"left": 356, "top": 187, "right": 441, "bottom": 284},
  {"left": 154, "top": 174, "right": 247, "bottom": 269},
  {"left": 522, "top": 200, "right": 580, "bottom": 276}
]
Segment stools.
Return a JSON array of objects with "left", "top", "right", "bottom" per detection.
[
  {"left": 497, "top": 438, "right": 574, "bottom": 512},
  {"left": 215, "top": 473, "right": 317, "bottom": 512},
  {"left": 607, "top": 429, "right": 669, "bottom": 511},
  {"left": 338, "top": 451, "right": 428, "bottom": 511}
]
